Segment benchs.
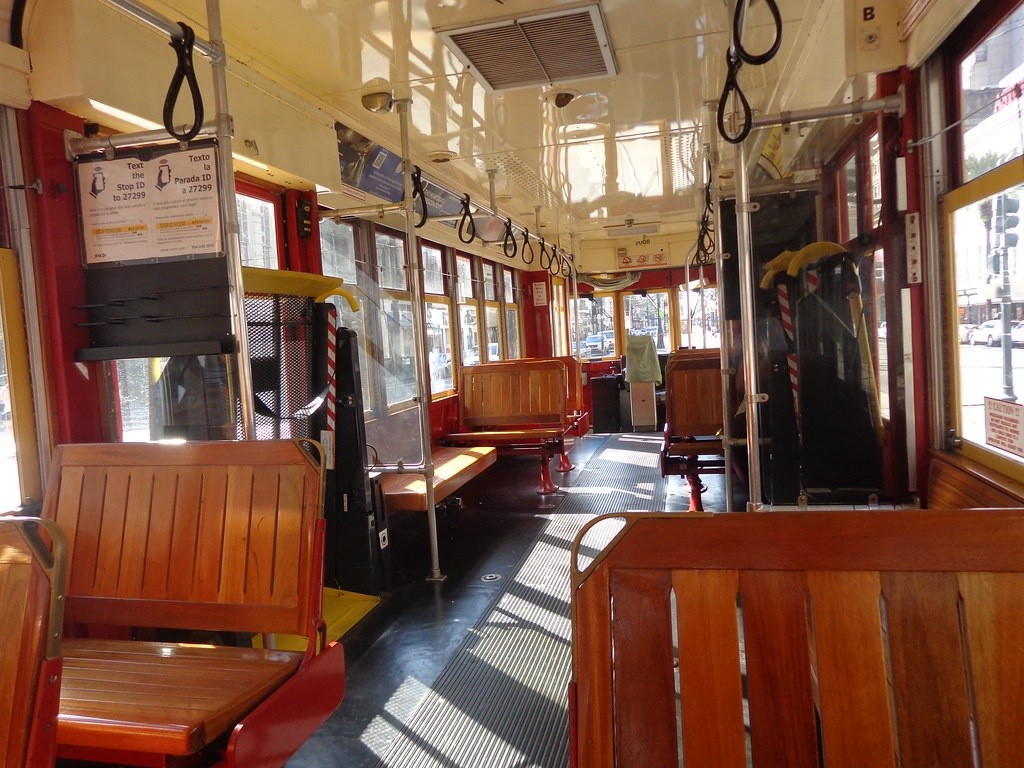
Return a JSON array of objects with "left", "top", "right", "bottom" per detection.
[
  {"left": 368, "top": 354, "right": 589, "bottom": 514},
  {"left": 0, "top": 514, "right": 68, "bottom": 768},
  {"left": 38, "top": 437, "right": 346, "bottom": 768},
  {"left": 568, "top": 503, "right": 1024, "bottom": 768},
  {"left": 805, "top": 450, "right": 1024, "bottom": 768},
  {"left": 659, "top": 348, "right": 763, "bottom": 511}
]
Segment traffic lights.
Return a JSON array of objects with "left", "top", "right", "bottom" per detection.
[
  {"left": 993, "top": 194, "right": 1019, "bottom": 249},
  {"left": 985, "top": 252, "right": 1002, "bottom": 274}
]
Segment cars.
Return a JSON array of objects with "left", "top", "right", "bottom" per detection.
[
  {"left": 429, "top": 352, "right": 452, "bottom": 381},
  {"left": 1010, "top": 319, "right": 1024, "bottom": 349},
  {"left": 571, "top": 339, "right": 592, "bottom": 359},
  {"left": 631, "top": 325, "right": 657, "bottom": 338},
  {"left": 0, "top": 374, "right": 11, "bottom": 420},
  {"left": 967, "top": 320, "right": 1021, "bottom": 347},
  {"left": 958, "top": 324, "right": 979, "bottom": 345},
  {"left": 464, "top": 342, "right": 499, "bottom": 365},
  {"left": 877, "top": 320, "right": 887, "bottom": 339}
]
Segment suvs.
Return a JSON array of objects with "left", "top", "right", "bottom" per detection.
[
  {"left": 585, "top": 333, "right": 610, "bottom": 356},
  {"left": 597, "top": 329, "right": 615, "bottom": 350}
]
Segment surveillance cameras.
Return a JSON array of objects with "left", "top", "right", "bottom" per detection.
[{"left": 543, "top": 91, "right": 575, "bottom": 109}]
